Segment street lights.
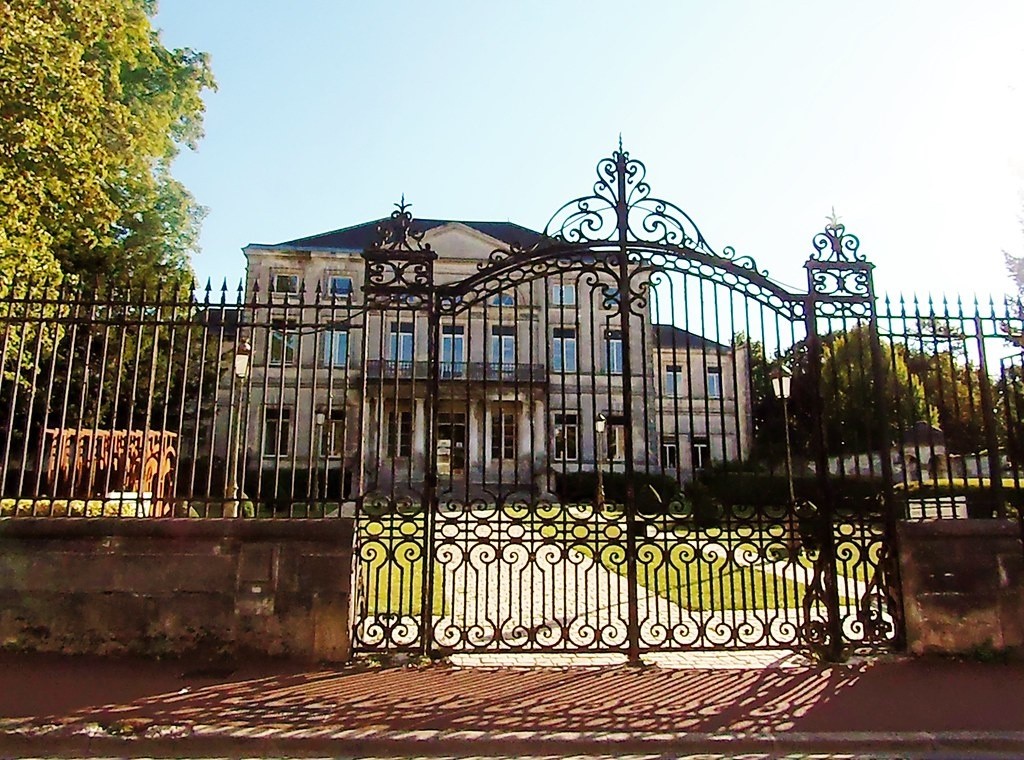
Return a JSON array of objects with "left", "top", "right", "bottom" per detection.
[
  {"left": 766, "top": 362, "right": 803, "bottom": 555},
  {"left": 223, "top": 336, "right": 254, "bottom": 518},
  {"left": 310, "top": 407, "right": 327, "bottom": 512},
  {"left": 594, "top": 413, "right": 608, "bottom": 513}
]
